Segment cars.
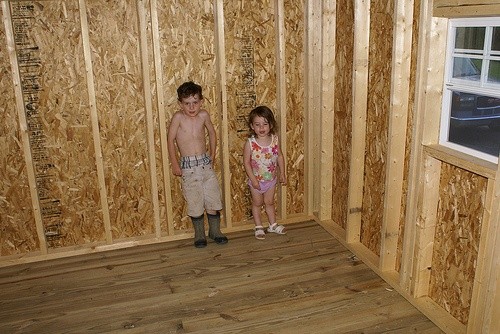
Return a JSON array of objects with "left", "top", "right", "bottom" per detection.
[{"left": 450, "top": 51, "right": 500, "bottom": 133}]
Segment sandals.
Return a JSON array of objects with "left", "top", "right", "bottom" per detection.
[
  {"left": 267, "top": 222, "right": 288, "bottom": 234},
  {"left": 254, "top": 226, "right": 266, "bottom": 239}
]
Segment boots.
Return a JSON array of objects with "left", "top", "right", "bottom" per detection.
[
  {"left": 207, "top": 211, "right": 227, "bottom": 243},
  {"left": 191, "top": 213, "right": 206, "bottom": 247}
]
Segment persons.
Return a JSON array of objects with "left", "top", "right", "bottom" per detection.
[
  {"left": 244, "top": 106, "right": 288, "bottom": 240},
  {"left": 167, "top": 81, "right": 228, "bottom": 248}
]
